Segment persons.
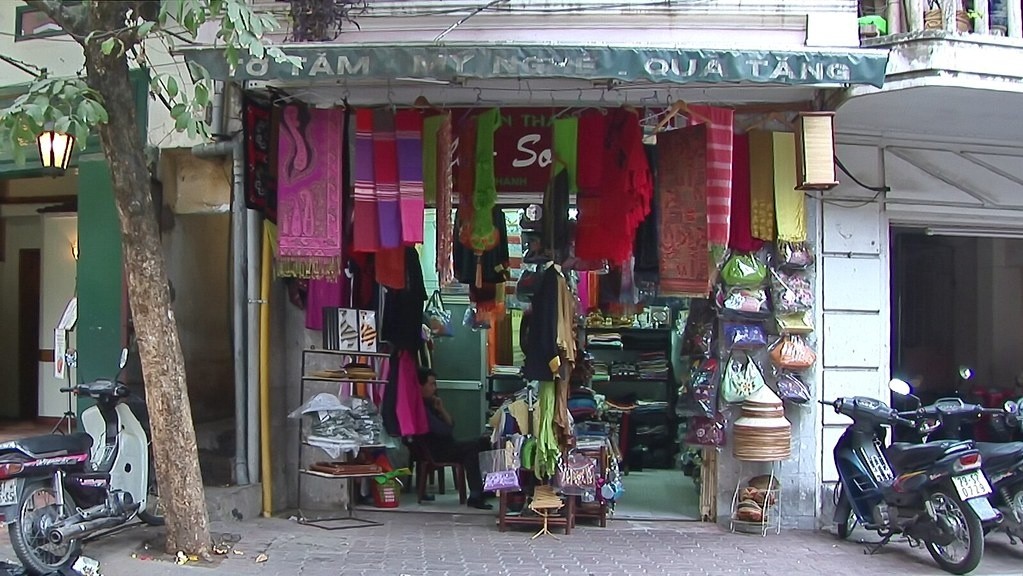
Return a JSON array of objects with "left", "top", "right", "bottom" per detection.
[{"left": 409, "top": 365, "right": 504, "bottom": 509}]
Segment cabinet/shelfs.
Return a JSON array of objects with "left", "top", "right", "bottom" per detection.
[
  {"left": 498, "top": 437, "right": 607, "bottom": 535},
  {"left": 587, "top": 325, "right": 677, "bottom": 468},
  {"left": 297, "top": 349, "right": 392, "bottom": 531}
]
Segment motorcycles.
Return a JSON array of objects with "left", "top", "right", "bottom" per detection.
[
  {"left": 0, "top": 347, "right": 165, "bottom": 576},
  {"left": 888, "top": 377, "right": 1023, "bottom": 546},
  {"left": 818, "top": 394, "right": 1004, "bottom": 575}
]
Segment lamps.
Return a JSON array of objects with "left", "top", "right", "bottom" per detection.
[
  {"left": 35, "top": 128, "right": 75, "bottom": 179},
  {"left": 790, "top": 112, "right": 839, "bottom": 192}
]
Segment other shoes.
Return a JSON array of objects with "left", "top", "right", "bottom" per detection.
[{"left": 467, "top": 498, "right": 493, "bottom": 509}]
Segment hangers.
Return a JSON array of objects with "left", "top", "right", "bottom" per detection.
[{"left": 269, "top": 78, "right": 794, "bottom": 137}]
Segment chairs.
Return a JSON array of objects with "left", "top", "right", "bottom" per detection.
[{"left": 399, "top": 433, "right": 467, "bottom": 504}]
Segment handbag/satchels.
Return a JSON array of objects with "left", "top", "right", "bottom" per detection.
[
  {"left": 423, "top": 290, "right": 453, "bottom": 336},
  {"left": 462, "top": 306, "right": 491, "bottom": 331},
  {"left": 678, "top": 240, "right": 818, "bottom": 447},
  {"left": 484, "top": 471, "right": 521, "bottom": 493},
  {"left": 517, "top": 210, "right": 548, "bottom": 356}
]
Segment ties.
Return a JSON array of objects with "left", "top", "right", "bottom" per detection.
[
  {"left": 360, "top": 312, "right": 376, "bottom": 349},
  {"left": 339, "top": 310, "right": 359, "bottom": 350}
]
